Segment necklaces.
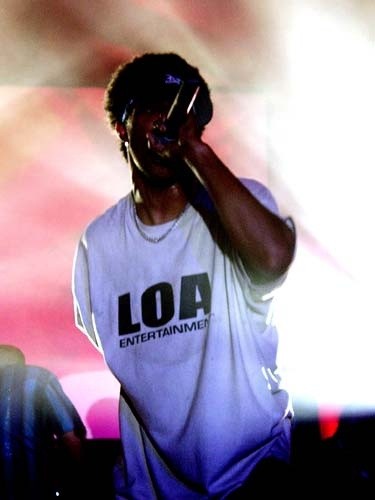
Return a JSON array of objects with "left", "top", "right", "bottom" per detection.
[{"left": 132, "top": 198, "right": 193, "bottom": 243}]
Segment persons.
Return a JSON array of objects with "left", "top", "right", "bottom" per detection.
[
  {"left": 72, "top": 52, "right": 295, "bottom": 500},
  {"left": 0, "top": 345, "right": 86, "bottom": 500}
]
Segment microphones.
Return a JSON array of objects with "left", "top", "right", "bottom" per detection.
[{"left": 158, "top": 82, "right": 200, "bottom": 142}]
